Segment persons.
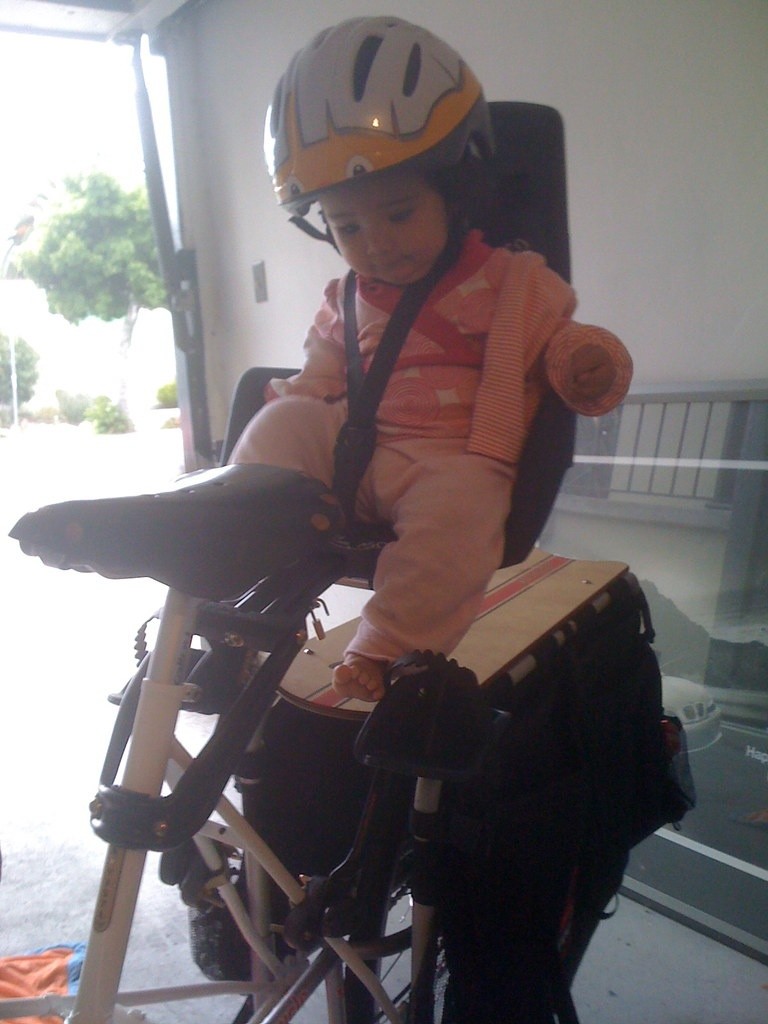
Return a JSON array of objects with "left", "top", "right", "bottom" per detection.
[{"left": 220, "top": 14, "right": 631, "bottom": 700}]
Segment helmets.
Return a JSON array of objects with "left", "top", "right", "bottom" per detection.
[{"left": 261, "top": 17, "right": 482, "bottom": 206}]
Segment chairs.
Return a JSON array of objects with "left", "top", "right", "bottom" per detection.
[{"left": 214, "top": 100, "right": 573, "bottom": 583}]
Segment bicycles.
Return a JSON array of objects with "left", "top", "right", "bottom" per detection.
[{"left": 0, "top": 464, "right": 585, "bottom": 1022}]
